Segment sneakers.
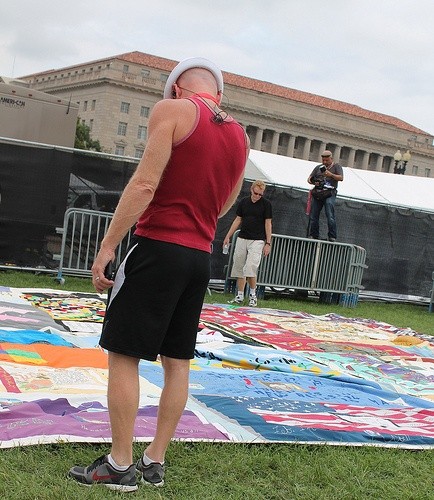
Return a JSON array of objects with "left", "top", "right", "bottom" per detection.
[
  {"left": 135, "top": 457, "right": 166, "bottom": 489},
  {"left": 66, "top": 455, "right": 138, "bottom": 493}
]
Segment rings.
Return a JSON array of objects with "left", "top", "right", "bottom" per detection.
[{"left": 95, "top": 277, "right": 99, "bottom": 281}]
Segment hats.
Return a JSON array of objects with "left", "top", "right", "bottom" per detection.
[{"left": 164, "top": 57, "right": 224, "bottom": 107}]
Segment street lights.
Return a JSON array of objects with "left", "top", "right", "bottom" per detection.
[{"left": 392, "top": 148, "right": 411, "bottom": 175}]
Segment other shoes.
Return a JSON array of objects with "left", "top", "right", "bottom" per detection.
[
  {"left": 308, "top": 236, "right": 313, "bottom": 239},
  {"left": 248, "top": 293, "right": 258, "bottom": 307},
  {"left": 227, "top": 294, "right": 244, "bottom": 304},
  {"left": 328, "top": 238, "right": 336, "bottom": 242}
]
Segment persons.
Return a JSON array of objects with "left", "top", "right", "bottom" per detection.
[
  {"left": 307, "top": 151, "right": 343, "bottom": 242},
  {"left": 222, "top": 180, "right": 274, "bottom": 307},
  {"left": 66, "top": 57, "right": 252, "bottom": 493}
]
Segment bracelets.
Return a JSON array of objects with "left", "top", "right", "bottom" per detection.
[{"left": 266, "top": 243, "right": 272, "bottom": 246}]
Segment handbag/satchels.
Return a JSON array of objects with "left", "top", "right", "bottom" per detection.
[{"left": 311, "top": 183, "right": 332, "bottom": 200}]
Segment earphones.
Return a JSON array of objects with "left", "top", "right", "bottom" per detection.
[{"left": 173, "top": 91, "right": 175, "bottom": 96}]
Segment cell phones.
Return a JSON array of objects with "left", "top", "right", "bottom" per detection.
[{"left": 104, "top": 260, "right": 112, "bottom": 280}]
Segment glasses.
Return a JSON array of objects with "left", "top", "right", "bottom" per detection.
[
  {"left": 320, "top": 154, "right": 332, "bottom": 158},
  {"left": 252, "top": 188, "right": 264, "bottom": 197}
]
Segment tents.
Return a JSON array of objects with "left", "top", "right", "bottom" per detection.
[{"left": 244, "top": 148, "right": 434, "bottom": 213}]
